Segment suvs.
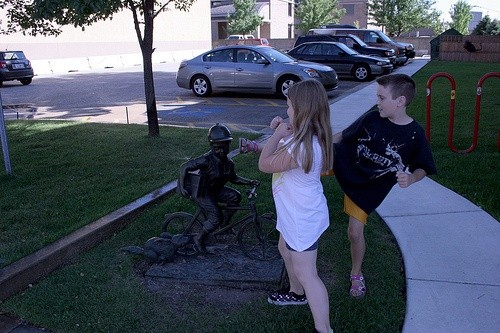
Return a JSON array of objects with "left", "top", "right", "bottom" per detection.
[{"left": 294, "top": 34, "right": 397, "bottom": 65}]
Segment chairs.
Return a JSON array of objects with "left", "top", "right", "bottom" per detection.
[
  {"left": 238, "top": 52, "right": 245, "bottom": 62},
  {"left": 247, "top": 53, "right": 254, "bottom": 60}
]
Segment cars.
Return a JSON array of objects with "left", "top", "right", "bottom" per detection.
[
  {"left": 175, "top": 45, "right": 339, "bottom": 101},
  {"left": 285, "top": 41, "right": 394, "bottom": 82},
  {"left": 0, "top": 50, "right": 34, "bottom": 86}
]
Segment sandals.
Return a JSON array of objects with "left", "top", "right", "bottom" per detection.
[
  {"left": 239, "top": 138, "right": 258, "bottom": 154},
  {"left": 350, "top": 273, "right": 366, "bottom": 299}
]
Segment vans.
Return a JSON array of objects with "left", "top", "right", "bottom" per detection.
[
  {"left": 227, "top": 35, "right": 255, "bottom": 39},
  {"left": 308, "top": 24, "right": 416, "bottom": 65},
  {"left": 235, "top": 38, "right": 269, "bottom": 46}
]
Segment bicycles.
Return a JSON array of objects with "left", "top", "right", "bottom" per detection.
[{"left": 161, "top": 181, "right": 282, "bottom": 261}]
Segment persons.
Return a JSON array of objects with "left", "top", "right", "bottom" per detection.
[
  {"left": 239, "top": 74, "right": 437, "bottom": 297},
  {"left": 258, "top": 77, "right": 347, "bottom": 333},
  {"left": 177, "top": 124, "right": 261, "bottom": 254}
]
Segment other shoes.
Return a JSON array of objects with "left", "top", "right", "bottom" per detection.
[{"left": 268, "top": 290, "right": 307, "bottom": 305}]
